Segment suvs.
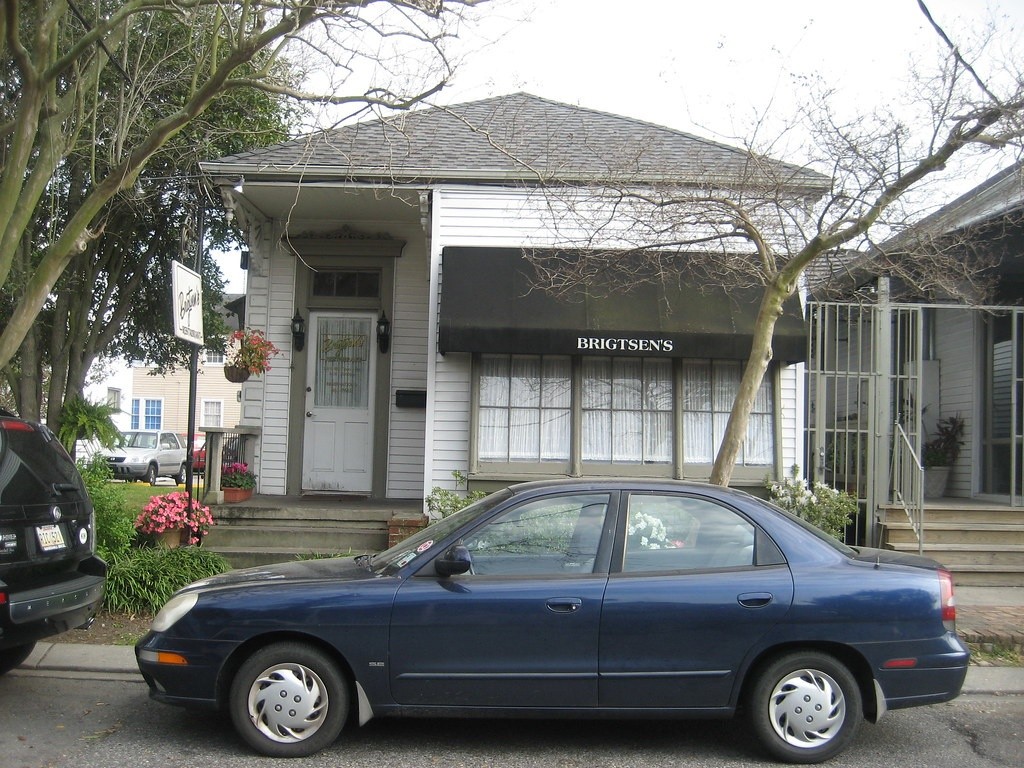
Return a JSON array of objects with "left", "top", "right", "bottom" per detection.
[
  {"left": 87, "top": 429, "right": 189, "bottom": 486},
  {"left": 0, "top": 406, "right": 107, "bottom": 676}
]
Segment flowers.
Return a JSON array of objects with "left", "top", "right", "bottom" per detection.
[
  {"left": 134, "top": 492, "right": 213, "bottom": 544},
  {"left": 221, "top": 462, "right": 258, "bottom": 491},
  {"left": 229, "top": 327, "right": 281, "bottom": 378}
]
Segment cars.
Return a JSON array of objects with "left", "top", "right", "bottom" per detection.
[
  {"left": 133, "top": 476, "right": 969, "bottom": 763},
  {"left": 191, "top": 434, "right": 239, "bottom": 478}
]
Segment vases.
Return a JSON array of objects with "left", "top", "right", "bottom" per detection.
[
  {"left": 221, "top": 487, "right": 253, "bottom": 502},
  {"left": 154, "top": 528, "right": 181, "bottom": 550},
  {"left": 224, "top": 365, "right": 250, "bottom": 383}
]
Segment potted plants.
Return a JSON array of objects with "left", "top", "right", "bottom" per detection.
[{"left": 921, "top": 402, "right": 965, "bottom": 499}]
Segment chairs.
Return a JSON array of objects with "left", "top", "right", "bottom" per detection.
[
  {"left": 707, "top": 532, "right": 753, "bottom": 568},
  {"left": 569, "top": 523, "right": 602, "bottom": 575}
]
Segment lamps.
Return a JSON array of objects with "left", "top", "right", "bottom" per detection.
[
  {"left": 376, "top": 310, "right": 391, "bottom": 354},
  {"left": 290, "top": 307, "right": 305, "bottom": 352}
]
